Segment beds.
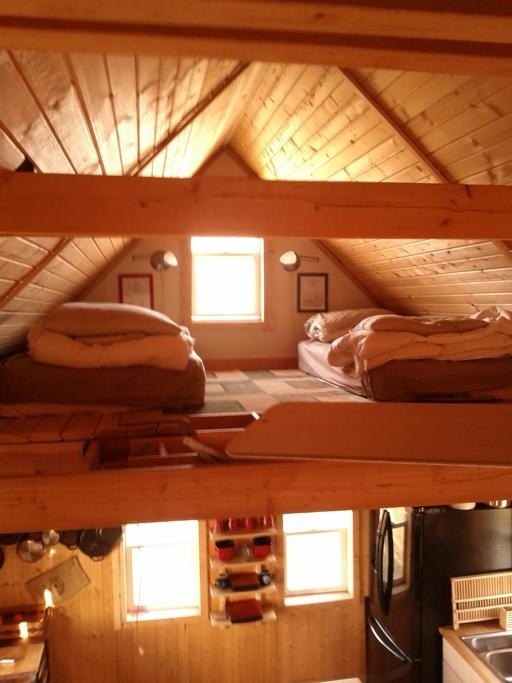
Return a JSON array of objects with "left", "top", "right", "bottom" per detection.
[
  {"left": 1, "top": 301, "right": 207, "bottom": 418},
  {"left": 298, "top": 308, "right": 512, "bottom": 400}
]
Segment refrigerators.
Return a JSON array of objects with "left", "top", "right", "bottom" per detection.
[{"left": 364, "top": 506, "right": 512, "bottom": 683}]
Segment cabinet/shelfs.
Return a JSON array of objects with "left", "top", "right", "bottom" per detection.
[{"left": 209, "top": 528, "right": 278, "bottom": 627}]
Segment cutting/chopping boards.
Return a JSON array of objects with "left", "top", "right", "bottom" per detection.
[{"left": 0, "top": 642, "right": 45, "bottom": 679}]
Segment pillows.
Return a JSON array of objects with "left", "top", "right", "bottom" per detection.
[
  {"left": 306, "top": 308, "right": 395, "bottom": 343},
  {"left": 349, "top": 313, "right": 488, "bottom": 335},
  {"left": 42, "top": 301, "right": 184, "bottom": 337}
]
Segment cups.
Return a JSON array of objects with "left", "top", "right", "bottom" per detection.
[{"left": 241, "top": 547, "right": 252, "bottom": 561}]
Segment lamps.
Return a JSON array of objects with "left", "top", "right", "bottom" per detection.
[
  {"left": 132, "top": 250, "right": 178, "bottom": 271},
  {"left": 279, "top": 249, "right": 320, "bottom": 271}
]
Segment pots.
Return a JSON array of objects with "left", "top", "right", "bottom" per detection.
[
  {"left": 96, "top": 527, "right": 122, "bottom": 545},
  {"left": 16, "top": 532, "right": 44, "bottom": 563},
  {"left": 61, "top": 530, "right": 78, "bottom": 551},
  {"left": 78, "top": 528, "right": 109, "bottom": 562},
  {"left": 41, "top": 530, "right": 61, "bottom": 546}
]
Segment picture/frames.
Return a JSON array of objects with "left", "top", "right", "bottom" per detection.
[
  {"left": 119, "top": 273, "right": 154, "bottom": 309},
  {"left": 297, "top": 273, "right": 329, "bottom": 312}
]
